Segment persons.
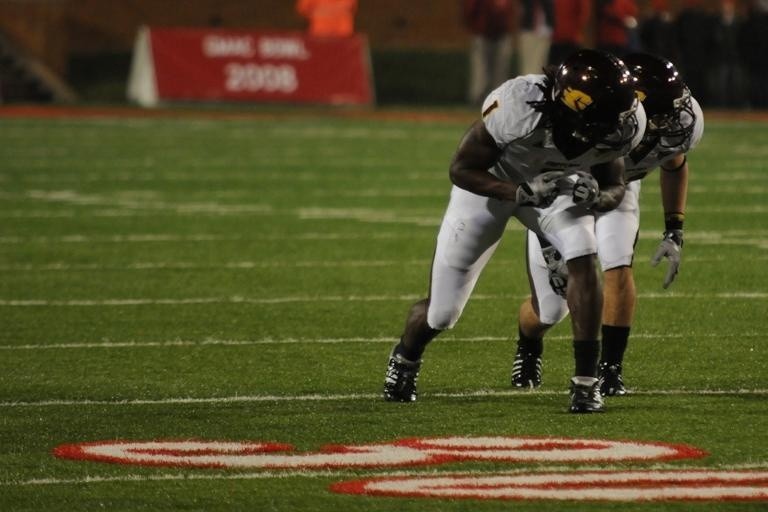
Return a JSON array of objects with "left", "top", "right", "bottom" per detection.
[
  {"left": 511, "top": 52, "right": 705, "bottom": 394},
  {"left": 384, "top": 50, "right": 646, "bottom": 414},
  {"left": 295, "top": 0, "right": 359, "bottom": 38}
]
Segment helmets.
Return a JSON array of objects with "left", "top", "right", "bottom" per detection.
[
  {"left": 620, "top": 50, "right": 696, "bottom": 154},
  {"left": 545, "top": 48, "right": 638, "bottom": 156}
]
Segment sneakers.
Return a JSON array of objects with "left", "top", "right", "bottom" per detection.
[
  {"left": 567, "top": 375, "right": 603, "bottom": 413},
  {"left": 383, "top": 344, "right": 423, "bottom": 402},
  {"left": 511, "top": 346, "right": 542, "bottom": 391},
  {"left": 596, "top": 359, "right": 627, "bottom": 397}
]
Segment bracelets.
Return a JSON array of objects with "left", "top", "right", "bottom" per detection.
[{"left": 665, "top": 212, "right": 685, "bottom": 230}]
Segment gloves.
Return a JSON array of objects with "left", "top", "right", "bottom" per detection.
[
  {"left": 512, "top": 170, "right": 565, "bottom": 209},
  {"left": 540, "top": 245, "right": 569, "bottom": 300},
  {"left": 649, "top": 228, "right": 683, "bottom": 289},
  {"left": 571, "top": 170, "right": 600, "bottom": 208}
]
